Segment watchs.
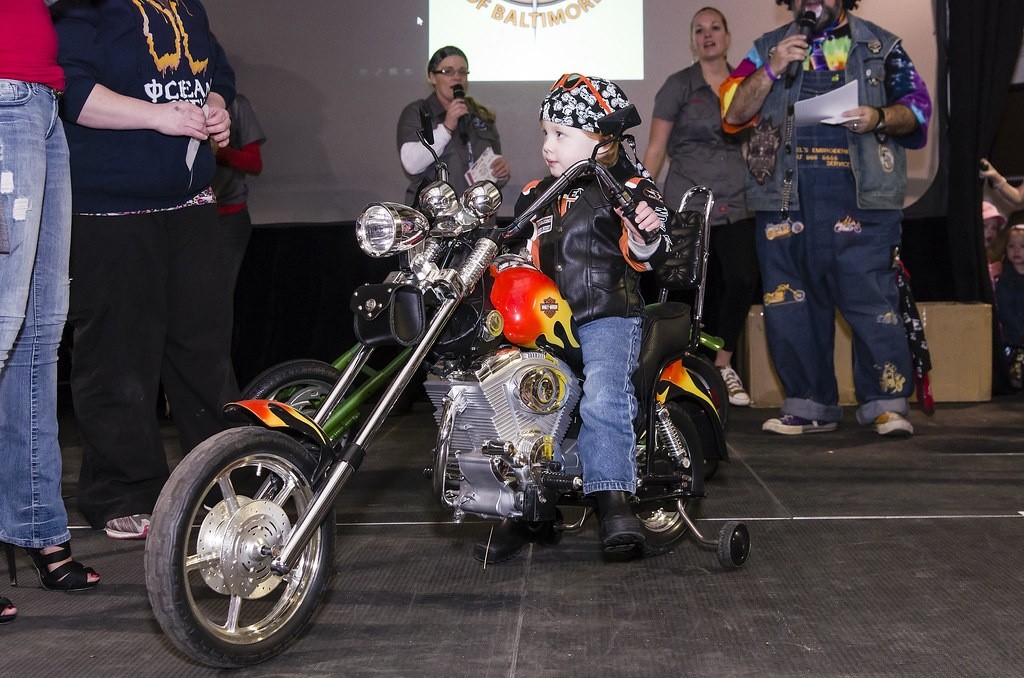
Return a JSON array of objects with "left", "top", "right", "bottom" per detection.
[{"left": 874, "top": 108, "right": 885, "bottom": 131}]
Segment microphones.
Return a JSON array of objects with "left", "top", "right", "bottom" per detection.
[
  {"left": 453, "top": 84, "right": 469, "bottom": 139},
  {"left": 784, "top": 10, "right": 817, "bottom": 89}
]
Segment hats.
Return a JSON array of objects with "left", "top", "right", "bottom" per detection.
[{"left": 982, "top": 199, "right": 1007, "bottom": 229}]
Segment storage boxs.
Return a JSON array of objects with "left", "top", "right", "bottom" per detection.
[{"left": 736, "top": 300, "right": 992, "bottom": 408}]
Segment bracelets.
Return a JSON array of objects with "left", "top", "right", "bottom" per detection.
[
  {"left": 444, "top": 124, "right": 454, "bottom": 133},
  {"left": 765, "top": 62, "right": 781, "bottom": 81},
  {"left": 994, "top": 177, "right": 1006, "bottom": 191}
]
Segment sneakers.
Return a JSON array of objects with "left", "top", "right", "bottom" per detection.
[
  {"left": 102, "top": 514, "right": 151, "bottom": 539},
  {"left": 716, "top": 365, "right": 750, "bottom": 405},
  {"left": 870, "top": 410, "right": 914, "bottom": 436},
  {"left": 762, "top": 414, "right": 837, "bottom": 434}
]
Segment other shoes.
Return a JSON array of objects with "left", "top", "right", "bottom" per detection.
[
  {"left": 472, "top": 506, "right": 563, "bottom": 563},
  {"left": 593, "top": 491, "right": 648, "bottom": 562}
]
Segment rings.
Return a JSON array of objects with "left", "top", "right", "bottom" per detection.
[{"left": 853, "top": 123, "right": 858, "bottom": 130}]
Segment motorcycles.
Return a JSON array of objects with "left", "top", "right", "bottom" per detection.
[{"left": 138, "top": 108, "right": 753, "bottom": 673}]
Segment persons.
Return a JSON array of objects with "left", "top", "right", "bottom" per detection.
[
  {"left": 641, "top": 8, "right": 757, "bottom": 408},
  {"left": 980, "top": 159, "right": 1024, "bottom": 391},
  {"left": 48, "top": 0, "right": 269, "bottom": 538},
  {"left": 719, "top": 0, "right": 932, "bottom": 436},
  {"left": 475, "top": 73, "right": 671, "bottom": 565},
  {"left": 0, "top": 0, "right": 100, "bottom": 624},
  {"left": 381, "top": 46, "right": 511, "bottom": 415}
]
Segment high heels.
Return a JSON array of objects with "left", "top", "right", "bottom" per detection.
[
  {"left": 0, "top": 597, "right": 17, "bottom": 625},
  {"left": 0, "top": 540, "right": 99, "bottom": 591}
]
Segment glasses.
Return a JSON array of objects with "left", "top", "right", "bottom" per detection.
[
  {"left": 550, "top": 73, "right": 611, "bottom": 114},
  {"left": 432, "top": 69, "right": 469, "bottom": 77}
]
